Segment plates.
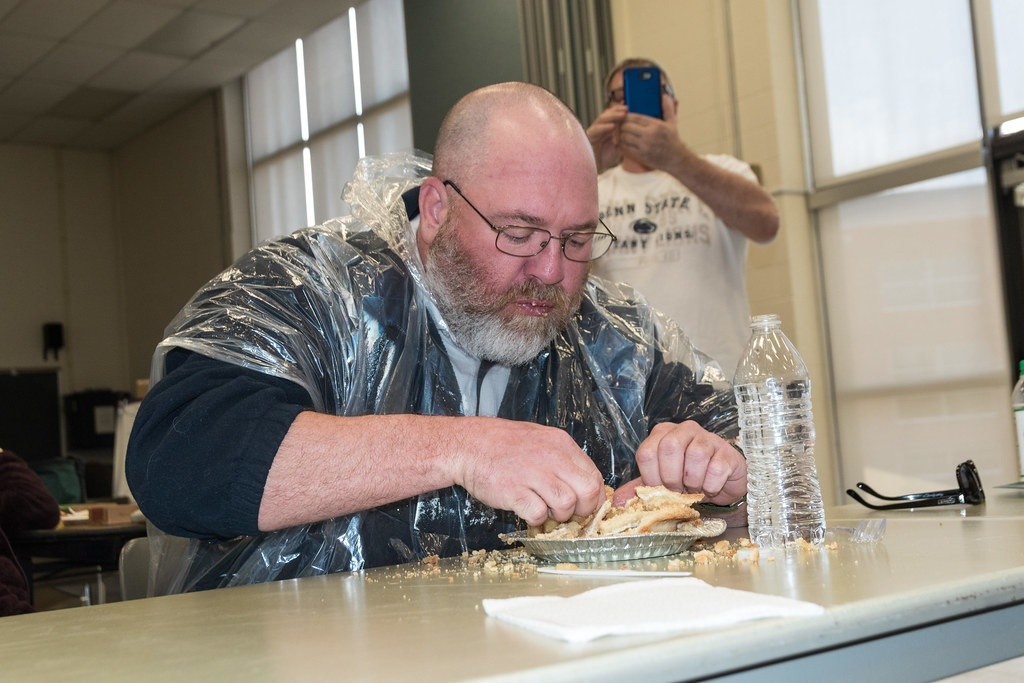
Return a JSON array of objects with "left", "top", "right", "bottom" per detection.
[
  {"left": 502, "top": 517, "right": 727, "bottom": 562},
  {"left": 60, "top": 511, "right": 89, "bottom": 524}
]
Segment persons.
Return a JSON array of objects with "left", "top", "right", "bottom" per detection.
[
  {"left": 122, "top": 82, "right": 753, "bottom": 595},
  {"left": 0, "top": 451, "right": 61, "bottom": 617},
  {"left": 586, "top": 56, "right": 780, "bottom": 384}
]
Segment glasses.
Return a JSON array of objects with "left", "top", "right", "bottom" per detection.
[
  {"left": 606, "top": 82, "right": 679, "bottom": 108},
  {"left": 846, "top": 459, "right": 988, "bottom": 511},
  {"left": 441, "top": 178, "right": 616, "bottom": 263}
]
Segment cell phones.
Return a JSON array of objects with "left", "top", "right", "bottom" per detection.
[{"left": 623, "top": 65, "right": 664, "bottom": 121}]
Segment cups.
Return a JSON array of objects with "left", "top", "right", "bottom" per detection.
[{"left": 89, "top": 508, "right": 108, "bottom": 525}]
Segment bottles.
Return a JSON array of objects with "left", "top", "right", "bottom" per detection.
[
  {"left": 1011, "top": 360, "right": 1024, "bottom": 484},
  {"left": 733, "top": 313, "right": 827, "bottom": 554}
]
